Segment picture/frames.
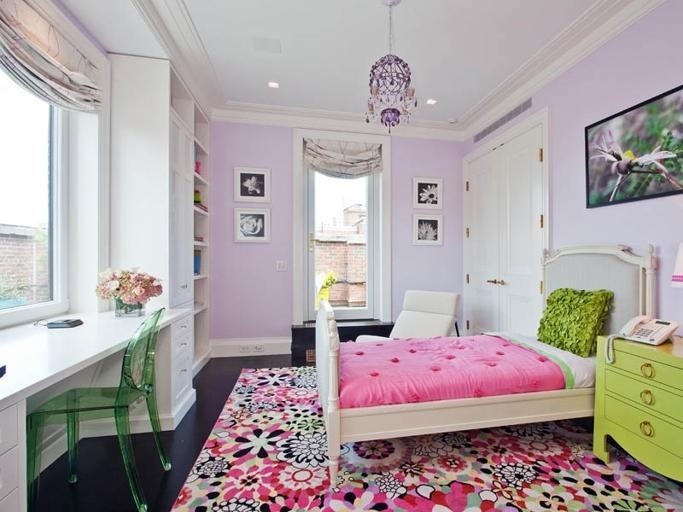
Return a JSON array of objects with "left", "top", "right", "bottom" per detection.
[
  {"left": 234, "top": 167, "right": 269, "bottom": 203},
  {"left": 412, "top": 177, "right": 442, "bottom": 209},
  {"left": 234, "top": 209, "right": 270, "bottom": 244},
  {"left": 585, "top": 86, "right": 683, "bottom": 208},
  {"left": 412, "top": 217, "right": 443, "bottom": 246}
]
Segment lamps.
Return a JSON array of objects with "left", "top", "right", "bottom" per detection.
[
  {"left": 366, "top": 0, "right": 417, "bottom": 133},
  {"left": 672, "top": 243, "right": 683, "bottom": 291}
]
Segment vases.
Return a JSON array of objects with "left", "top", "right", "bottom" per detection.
[{"left": 116, "top": 299, "right": 143, "bottom": 317}]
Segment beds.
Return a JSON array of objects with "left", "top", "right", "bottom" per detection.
[{"left": 316, "top": 244, "right": 657, "bottom": 493}]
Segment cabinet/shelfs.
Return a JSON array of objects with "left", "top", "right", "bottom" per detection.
[
  {"left": 110, "top": 53, "right": 210, "bottom": 431},
  {"left": 591, "top": 335, "right": 683, "bottom": 486}
]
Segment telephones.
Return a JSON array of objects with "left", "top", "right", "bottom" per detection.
[{"left": 620, "top": 315, "right": 679, "bottom": 346}]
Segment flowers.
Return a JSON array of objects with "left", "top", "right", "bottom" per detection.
[{"left": 98, "top": 273, "right": 162, "bottom": 305}]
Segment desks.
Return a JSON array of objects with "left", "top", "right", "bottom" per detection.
[
  {"left": 292, "top": 321, "right": 394, "bottom": 365},
  {"left": 0, "top": 308, "right": 189, "bottom": 511}
]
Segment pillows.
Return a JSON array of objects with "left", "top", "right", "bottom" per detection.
[{"left": 538, "top": 288, "right": 613, "bottom": 357}]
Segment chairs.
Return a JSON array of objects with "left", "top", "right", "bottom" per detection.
[
  {"left": 27, "top": 309, "right": 171, "bottom": 511},
  {"left": 356, "top": 291, "right": 461, "bottom": 344}
]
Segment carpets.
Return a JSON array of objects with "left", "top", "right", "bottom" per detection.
[{"left": 170, "top": 366, "right": 682, "bottom": 512}]
